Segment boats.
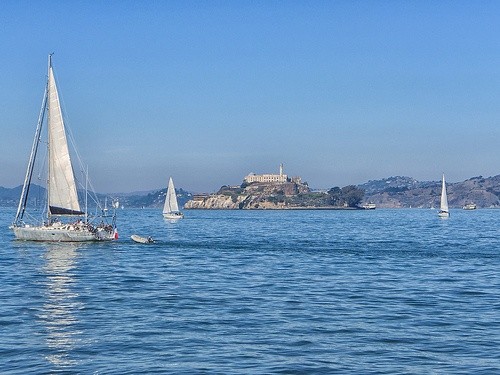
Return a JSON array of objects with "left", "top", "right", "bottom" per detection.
[
  {"left": 463, "top": 203, "right": 476, "bottom": 210},
  {"left": 363, "top": 203, "right": 375, "bottom": 209}
]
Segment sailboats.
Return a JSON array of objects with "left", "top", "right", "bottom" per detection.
[
  {"left": 438, "top": 174, "right": 450, "bottom": 217},
  {"left": 162, "top": 176, "right": 184, "bottom": 219},
  {"left": 9, "top": 53, "right": 118, "bottom": 241}
]
